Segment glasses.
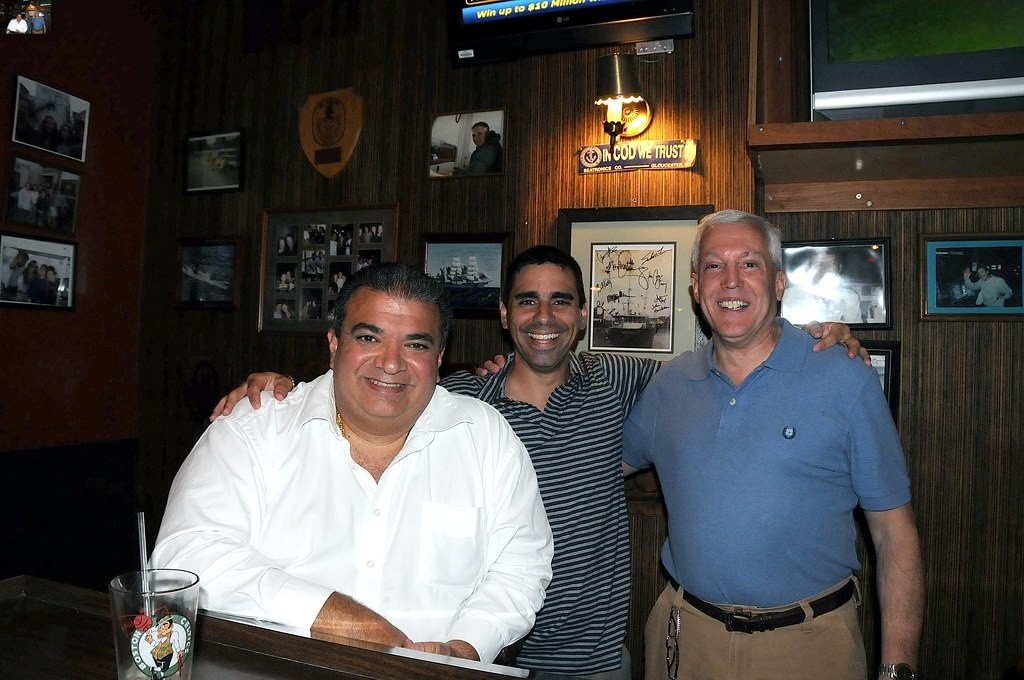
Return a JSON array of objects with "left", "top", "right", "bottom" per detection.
[{"left": 666, "top": 607, "right": 681, "bottom": 680}]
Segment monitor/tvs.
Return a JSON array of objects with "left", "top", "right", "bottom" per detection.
[{"left": 445, "top": 0, "right": 695, "bottom": 68}]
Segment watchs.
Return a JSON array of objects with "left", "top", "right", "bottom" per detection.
[{"left": 878, "top": 663, "right": 915, "bottom": 680}]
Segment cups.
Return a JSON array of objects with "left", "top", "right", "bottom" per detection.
[{"left": 109, "top": 569, "right": 199, "bottom": 680}]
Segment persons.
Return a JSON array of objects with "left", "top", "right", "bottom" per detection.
[
  {"left": 452, "top": 122, "right": 502, "bottom": 174},
  {"left": 16, "top": 182, "right": 66, "bottom": 230},
  {"left": 17, "top": 113, "right": 74, "bottom": 155},
  {"left": 963, "top": 265, "right": 1012, "bottom": 307},
  {"left": 6, "top": 11, "right": 46, "bottom": 34},
  {"left": 274, "top": 223, "right": 383, "bottom": 319},
  {"left": 147, "top": 262, "right": 554, "bottom": 663},
  {"left": 479, "top": 208, "right": 924, "bottom": 680},
  {"left": 209, "top": 245, "right": 872, "bottom": 680},
  {"left": 0, "top": 249, "right": 59, "bottom": 305},
  {"left": 781, "top": 248, "right": 862, "bottom": 324}
]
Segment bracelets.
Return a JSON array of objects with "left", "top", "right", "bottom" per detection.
[{"left": 284, "top": 374, "right": 295, "bottom": 390}]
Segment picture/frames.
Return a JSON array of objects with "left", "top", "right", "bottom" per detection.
[{"left": 0, "top": 75, "right": 1024, "bottom": 434}]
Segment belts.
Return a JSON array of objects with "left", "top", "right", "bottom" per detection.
[{"left": 657, "top": 559, "right": 854, "bottom": 634}]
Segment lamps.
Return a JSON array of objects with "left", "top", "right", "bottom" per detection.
[{"left": 583, "top": 45, "right": 653, "bottom": 158}]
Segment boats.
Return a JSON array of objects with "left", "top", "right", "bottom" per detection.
[{"left": 434, "top": 254, "right": 496, "bottom": 304}]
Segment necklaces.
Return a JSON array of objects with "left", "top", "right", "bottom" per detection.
[{"left": 336, "top": 408, "right": 345, "bottom": 438}]
